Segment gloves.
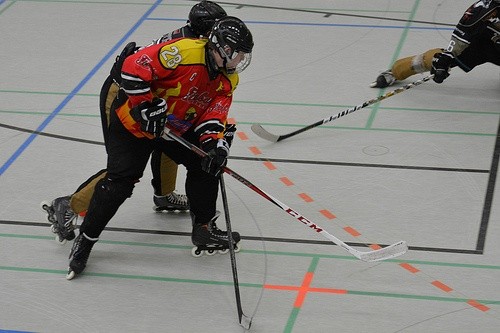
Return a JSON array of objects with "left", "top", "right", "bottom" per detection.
[
  {"left": 195, "top": 119, "right": 229, "bottom": 176},
  {"left": 430, "top": 52, "right": 452, "bottom": 83},
  {"left": 129, "top": 96, "right": 168, "bottom": 137},
  {"left": 224, "top": 123, "right": 236, "bottom": 149}
]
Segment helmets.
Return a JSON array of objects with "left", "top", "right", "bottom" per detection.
[
  {"left": 189, "top": 0, "right": 228, "bottom": 35},
  {"left": 209, "top": 16, "right": 254, "bottom": 74}
]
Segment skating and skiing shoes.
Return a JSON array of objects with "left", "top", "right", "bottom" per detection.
[
  {"left": 151, "top": 179, "right": 189, "bottom": 213},
  {"left": 66, "top": 232, "right": 99, "bottom": 280},
  {"left": 40, "top": 195, "right": 78, "bottom": 242},
  {"left": 370, "top": 68, "right": 397, "bottom": 88},
  {"left": 191, "top": 210, "right": 240, "bottom": 257}
]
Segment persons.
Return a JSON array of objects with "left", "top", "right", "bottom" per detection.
[
  {"left": 66, "top": 16, "right": 255, "bottom": 282},
  {"left": 40, "top": 0, "right": 228, "bottom": 244},
  {"left": 370, "top": 0, "right": 500, "bottom": 88}
]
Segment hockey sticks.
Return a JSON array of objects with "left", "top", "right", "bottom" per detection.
[
  {"left": 162, "top": 126, "right": 408, "bottom": 263},
  {"left": 219, "top": 172, "right": 253, "bottom": 329},
  {"left": 251, "top": 68, "right": 452, "bottom": 143}
]
